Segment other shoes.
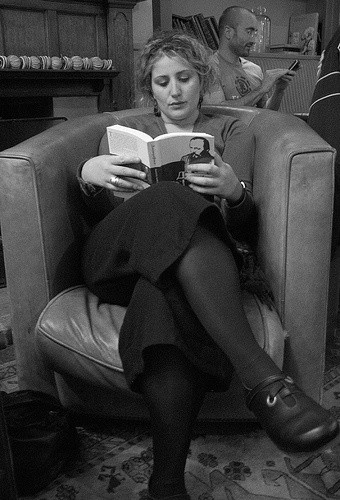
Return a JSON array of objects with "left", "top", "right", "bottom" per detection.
[{"left": 242, "top": 372, "right": 339, "bottom": 453}]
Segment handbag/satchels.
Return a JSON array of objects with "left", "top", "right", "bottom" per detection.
[{"left": 0, "top": 389, "right": 82, "bottom": 500}]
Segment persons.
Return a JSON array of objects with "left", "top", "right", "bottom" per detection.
[
  {"left": 76, "top": 29, "right": 340, "bottom": 500},
  {"left": 203, "top": 6, "right": 302, "bottom": 110}
]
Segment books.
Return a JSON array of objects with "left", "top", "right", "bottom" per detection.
[
  {"left": 107, "top": 123, "right": 215, "bottom": 203},
  {"left": 289, "top": 13, "right": 319, "bottom": 52},
  {"left": 172, "top": 13, "right": 219, "bottom": 50}
]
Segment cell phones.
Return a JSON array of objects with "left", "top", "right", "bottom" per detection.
[{"left": 288, "top": 59, "right": 300, "bottom": 71}]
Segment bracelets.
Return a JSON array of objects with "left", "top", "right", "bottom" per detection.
[{"left": 225, "top": 181, "right": 246, "bottom": 206}]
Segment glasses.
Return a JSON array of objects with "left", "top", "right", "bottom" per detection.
[{"left": 231, "top": 26, "right": 259, "bottom": 39}]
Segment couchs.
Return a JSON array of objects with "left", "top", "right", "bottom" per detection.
[{"left": 0, "top": 103, "right": 337, "bottom": 422}]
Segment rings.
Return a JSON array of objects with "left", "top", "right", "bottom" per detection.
[{"left": 109, "top": 175, "right": 119, "bottom": 185}]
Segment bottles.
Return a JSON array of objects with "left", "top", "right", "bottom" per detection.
[{"left": 250, "top": 6, "right": 271, "bottom": 53}]
